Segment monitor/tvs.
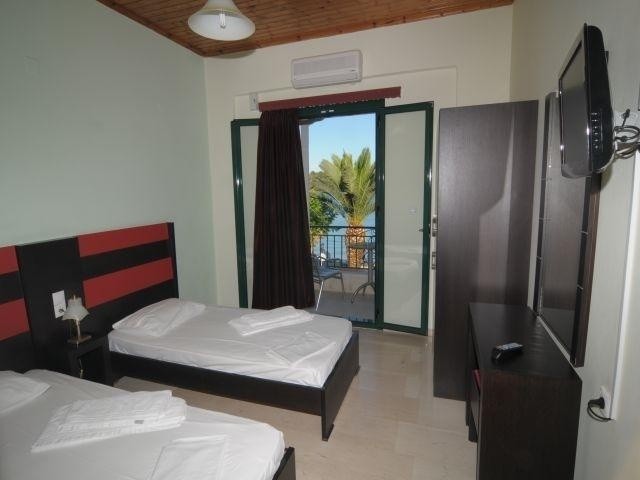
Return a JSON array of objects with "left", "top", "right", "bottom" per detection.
[{"left": 557, "top": 21, "right": 613, "bottom": 181}]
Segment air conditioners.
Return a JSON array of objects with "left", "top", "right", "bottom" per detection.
[{"left": 290, "top": 49, "right": 363, "bottom": 90}]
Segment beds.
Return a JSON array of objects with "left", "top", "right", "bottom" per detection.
[
  {"left": 0, "top": 245, "right": 296, "bottom": 480},
  {"left": 76, "top": 221, "right": 360, "bottom": 441}
]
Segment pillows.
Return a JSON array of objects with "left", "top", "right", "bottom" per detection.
[
  {"left": 0, "top": 369, "right": 52, "bottom": 418},
  {"left": 111, "top": 296, "right": 207, "bottom": 338}
]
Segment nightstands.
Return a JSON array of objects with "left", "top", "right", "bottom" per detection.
[{"left": 48, "top": 331, "right": 114, "bottom": 387}]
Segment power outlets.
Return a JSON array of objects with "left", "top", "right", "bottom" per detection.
[
  {"left": 50, "top": 289, "right": 67, "bottom": 319},
  {"left": 598, "top": 384, "right": 612, "bottom": 419}
]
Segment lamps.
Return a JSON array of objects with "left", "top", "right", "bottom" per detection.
[
  {"left": 187, "top": 0, "right": 257, "bottom": 42},
  {"left": 60, "top": 294, "right": 92, "bottom": 346}
]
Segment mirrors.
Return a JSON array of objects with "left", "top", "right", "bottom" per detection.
[{"left": 532, "top": 90, "right": 602, "bottom": 368}]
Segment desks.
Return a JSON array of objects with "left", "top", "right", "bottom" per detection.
[
  {"left": 463, "top": 302, "right": 583, "bottom": 480},
  {"left": 346, "top": 242, "right": 376, "bottom": 304}
]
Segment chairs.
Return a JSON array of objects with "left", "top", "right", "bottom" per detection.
[{"left": 310, "top": 252, "right": 345, "bottom": 312}]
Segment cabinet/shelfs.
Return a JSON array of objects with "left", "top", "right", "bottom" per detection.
[{"left": 433, "top": 98, "right": 539, "bottom": 401}]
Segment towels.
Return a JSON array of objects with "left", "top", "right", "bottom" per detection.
[
  {"left": 226, "top": 303, "right": 314, "bottom": 338},
  {"left": 28, "top": 388, "right": 188, "bottom": 454}
]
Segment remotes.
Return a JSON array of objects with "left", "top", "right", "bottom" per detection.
[{"left": 493, "top": 340, "right": 523, "bottom": 359}]
